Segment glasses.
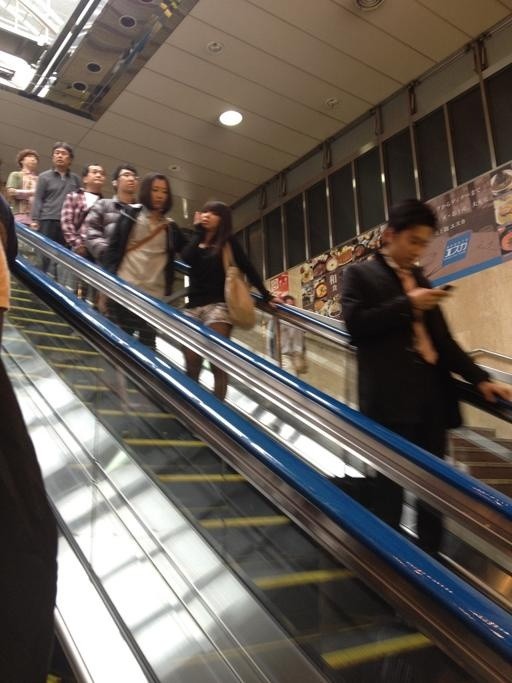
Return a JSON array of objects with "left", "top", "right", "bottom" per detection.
[{"left": 115, "top": 172, "right": 141, "bottom": 181}]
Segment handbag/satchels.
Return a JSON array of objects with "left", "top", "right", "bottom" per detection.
[
  {"left": 292, "top": 352, "right": 310, "bottom": 376},
  {"left": 224, "top": 264, "right": 257, "bottom": 331}
]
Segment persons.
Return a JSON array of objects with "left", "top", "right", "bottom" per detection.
[
  {"left": 29, "top": 142, "right": 82, "bottom": 281},
  {"left": 266, "top": 294, "right": 308, "bottom": 379},
  {"left": 59, "top": 161, "right": 106, "bottom": 305},
  {"left": 80, "top": 165, "right": 138, "bottom": 315},
  {"left": 339, "top": 194, "right": 511, "bottom": 558},
  {"left": 102, "top": 173, "right": 188, "bottom": 351},
  {"left": 179, "top": 201, "right": 285, "bottom": 402},
  {"left": 5, "top": 148, "right": 39, "bottom": 226}
]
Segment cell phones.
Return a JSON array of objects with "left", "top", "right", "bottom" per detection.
[{"left": 437, "top": 282, "right": 453, "bottom": 292}]
